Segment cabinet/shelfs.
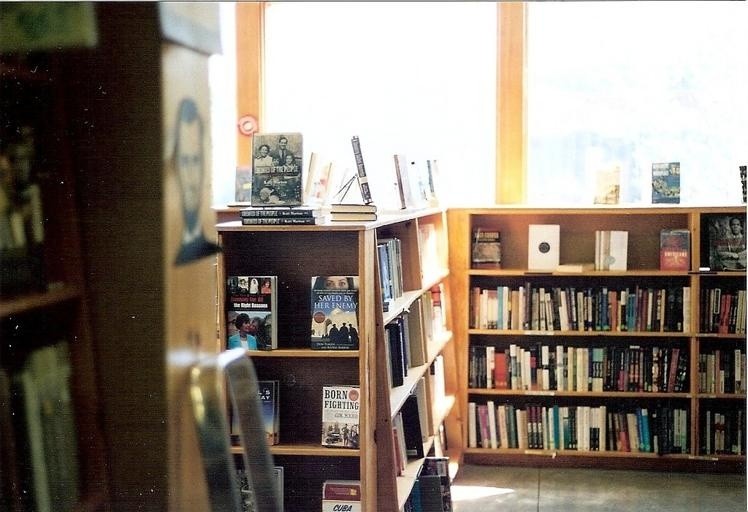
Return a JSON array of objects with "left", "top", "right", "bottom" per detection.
[
  {"left": 1, "top": 3, "right": 229, "bottom": 512},
  {"left": 218, "top": 222, "right": 376, "bottom": 512},
  {"left": 376, "top": 202, "right": 467, "bottom": 512},
  {"left": 445, "top": 206, "right": 747, "bottom": 474}
]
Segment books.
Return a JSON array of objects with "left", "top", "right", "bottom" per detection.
[
  {"left": 320, "top": 384, "right": 360, "bottom": 450},
  {"left": 697, "top": 350, "right": 745, "bottom": 395},
  {"left": 470, "top": 283, "right": 690, "bottom": 334},
  {"left": 469, "top": 343, "right": 688, "bottom": 392},
  {"left": 699, "top": 287, "right": 745, "bottom": 335},
  {"left": 649, "top": 160, "right": 679, "bottom": 205},
  {"left": 427, "top": 158, "right": 440, "bottom": 195},
  {"left": 321, "top": 481, "right": 361, "bottom": 512},
  {"left": 698, "top": 407, "right": 745, "bottom": 456},
  {"left": 224, "top": 272, "right": 277, "bottom": 350},
  {"left": 240, "top": 133, "right": 378, "bottom": 224},
  {"left": 593, "top": 165, "right": 620, "bottom": 206},
  {"left": 471, "top": 223, "right": 693, "bottom": 272},
  {"left": 1, "top": 130, "right": 98, "bottom": 511},
  {"left": 705, "top": 216, "right": 746, "bottom": 272},
  {"left": 375, "top": 223, "right": 454, "bottom": 512},
  {"left": 393, "top": 152, "right": 412, "bottom": 211},
  {"left": 309, "top": 275, "right": 358, "bottom": 350},
  {"left": 229, "top": 379, "right": 279, "bottom": 446},
  {"left": 470, "top": 402, "right": 689, "bottom": 456},
  {"left": 237, "top": 464, "right": 285, "bottom": 511}
]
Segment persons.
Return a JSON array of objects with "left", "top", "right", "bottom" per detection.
[{"left": 166, "top": 100, "right": 222, "bottom": 269}]
